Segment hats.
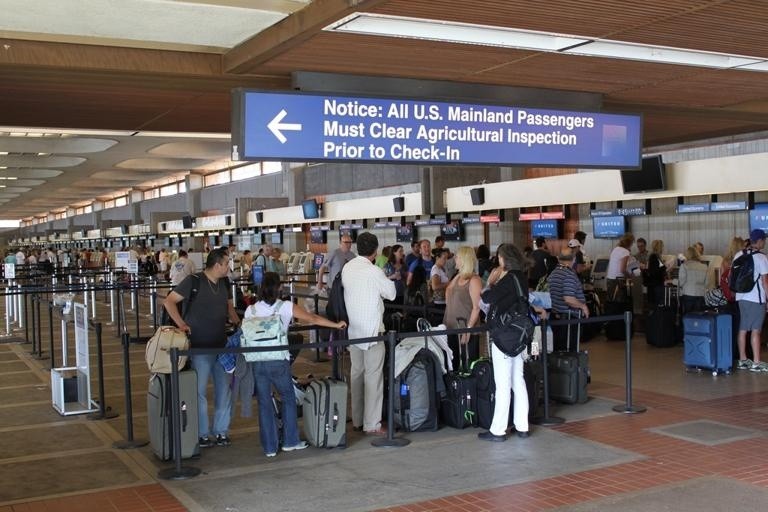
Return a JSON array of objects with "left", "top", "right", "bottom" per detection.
[
  {"left": 750, "top": 229, "right": 767, "bottom": 240},
  {"left": 568, "top": 239, "right": 584, "bottom": 247}
]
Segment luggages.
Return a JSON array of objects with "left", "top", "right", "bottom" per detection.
[
  {"left": 469, "top": 330, "right": 495, "bottom": 428},
  {"left": 308, "top": 285, "right": 328, "bottom": 352},
  {"left": 644, "top": 284, "right": 678, "bottom": 346},
  {"left": 607, "top": 277, "right": 634, "bottom": 340},
  {"left": 683, "top": 307, "right": 732, "bottom": 376},
  {"left": 524, "top": 360, "right": 546, "bottom": 420},
  {"left": 147, "top": 370, "right": 200, "bottom": 461},
  {"left": 546, "top": 309, "right": 591, "bottom": 405},
  {"left": 438, "top": 317, "right": 477, "bottom": 429},
  {"left": 393, "top": 321, "right": 437, "bottom": 431},
  {"left": 303, "top": 375, "right": 347, "bottom": 448}
]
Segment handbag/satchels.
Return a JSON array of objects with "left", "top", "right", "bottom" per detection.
[
  {"left": 720, "top": 267, "right": 735, "bottom": 303},
  {"left": 704, "top": 287, "right": 727, "bottom": 307},
  {"left": 534, "top": 326, "right": 553, "bottom": 356},
  {"left": 536, "top": 274, "right": 550, "bottom": 291},
  {"left": 145, "top": 326, "right": 189, "bottom": 373}
]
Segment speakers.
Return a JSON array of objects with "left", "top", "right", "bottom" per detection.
[
  {"left": 255, "top": 212, "right": 263, "bottom": 223},
  {"left": 224, "top": 216, "right": 231, "bottom": 225},
  {"left": 161, "top": 223, "right": 166, "bottom": 231},
  {"left": 470, "top": 188, "right": 482, "bottom": 205},
  {"left": 393, "top": 197, "right": 403, "bottom": 212}
]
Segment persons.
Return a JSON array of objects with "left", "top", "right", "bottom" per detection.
[{"left": 3, "top": 227, "right": 768, "bottom": 457}]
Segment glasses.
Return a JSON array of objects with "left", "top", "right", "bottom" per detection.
[{"left": 343, "top": 240, "right": 352, "bottom": 243}]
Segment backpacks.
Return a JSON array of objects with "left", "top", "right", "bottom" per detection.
[
  {"left": 249, "top": 254, "right": 266, "bottom": 278},
  {"left": 240, "top": 301, "right": 291, "bottom": 362},
  {"left": 486, "top": 275, "right": 535, "bottom": 357},
  {"left": 729, "top": 249, "right": 762, "bottom": 294},
  {"left": 161, "top": 274, "right": 200, "bottom": 326}
]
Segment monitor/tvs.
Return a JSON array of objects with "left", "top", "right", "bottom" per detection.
[
  {"left": 252, "top": 235, "right": 265, "bottom": 244},
  {"left": 120, "top": 224, "right": 127, "bottom": 234},
  {"left": 309, "top": 230, "right": 326, "bottom": 244},
  {"left": 338, "top": 228, "right": 357, "bottom": 243},
  {"left": 590, "top": 255, "right": 611, "bottom": 278},
  {"left": 208, "top": 235, "right": 215, "bottom": 245},
  {"left": 395, "top": 221, "right": 415, "bottom": 242},
  {"left": 592, "top": 215, "right": 627, "bottom": 239},
  {"left": 270, "top": 233, "right": 283, "bottom": 244},
  {"left": 221, "top": 235, "right": 232, "bottom": 246},
  {"left": 440, "top": 219, "right": 462, "bottom": 240},
  {"left": 620, "top": 154, "right": 666, "bottom": 194},
  {"left": 531, "top": 219, "right": 560, "bottom": 239},
  {"left": 301, "top": 199, "right": 319, "bottom": 219},
  {"left": 182, "top": 215, "right": 193, "bottom": 229},
  {"left": 748, "top": 201, "right": 768, "bottom": 238}
]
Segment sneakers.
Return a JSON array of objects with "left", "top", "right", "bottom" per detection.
[
  {"left": 216, "top": 434, "right": 233, "bottom": 446},
  {"left": 478, "top": 431, "right": 506, "bottom": 442},
  {"left": 281, "top": 441, "right": 310, "bottom": 451},
  {"left": 511, "top": 427, "right": 529, "bottom": 437},
  {"left": 201, "top": 436, "right": 213, "bottom": 448},
  {"left": 748, "top": 361, "right": 768, "bottom": 373},
  {"left": 266, "top": 453, "right": 276, "bottom": 457},
  {"left": 737, "top": 359, "right": 754, "bottom": 370}
]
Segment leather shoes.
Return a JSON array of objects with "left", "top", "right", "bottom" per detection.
[
  {"left": 364, "top": 428, "right": 386, "bottom": 436},
  {"left": 354, "top": 426, "right": 363, "bottom": 431}
]
